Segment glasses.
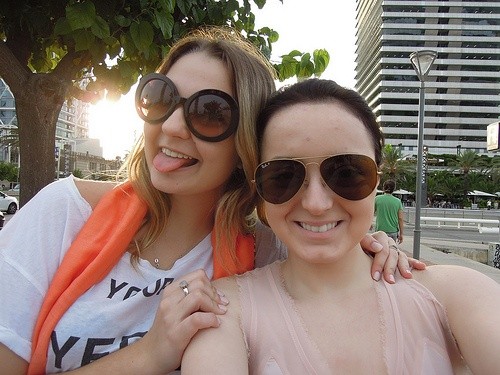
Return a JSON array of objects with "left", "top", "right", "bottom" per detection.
[
  {"left": 135, "top": 73, "right": 240, "bottom": 143},
  {"left": 250, "top": 152, "right": 383, "bottom": 206}
]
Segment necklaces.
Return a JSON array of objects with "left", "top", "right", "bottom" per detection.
[{"left": 154, "top": 228, "right": 213, "bottom": 268}]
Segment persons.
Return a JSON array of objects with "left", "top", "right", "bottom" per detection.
[
  {"left": 0, "top": 36, "right": 426, "bottom": 375},
  {"left": 180, "top": 79, "right": 500, "bottom": 375},
  {"left": 374, "top": 180, "right": 404, "bottom": 244}
]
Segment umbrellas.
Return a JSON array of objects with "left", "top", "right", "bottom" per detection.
[{"left": 376, "top": 188, "right": 497, "bottom": 197}]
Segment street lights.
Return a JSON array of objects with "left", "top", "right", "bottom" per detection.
[{"left": 409, "top": 48, "right": 437, "bottom": 260}]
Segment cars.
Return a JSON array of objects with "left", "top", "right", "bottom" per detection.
[{"left": 0, "top": 191, "right": 20, "bottom": 229}]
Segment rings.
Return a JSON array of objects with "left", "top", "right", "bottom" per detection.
[
  {"left": 179, "top": 281, "right": 189, "bottom": 295},
  {"left": 389, "top": 246, "right": 400, "bottom": 256}
]
